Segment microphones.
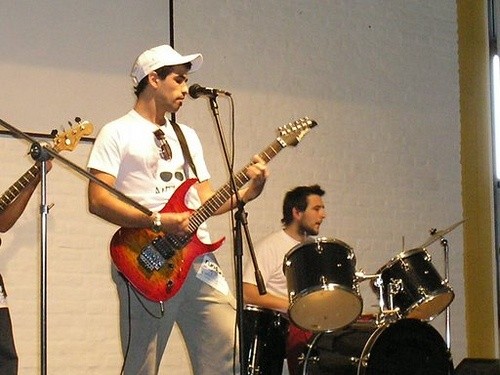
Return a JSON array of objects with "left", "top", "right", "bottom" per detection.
[
  {"left": 430, "top": 228, "right": 448, "bottom": 245},
  {"left": 189, "top": 83, "right": 230, "bottom": 99}
]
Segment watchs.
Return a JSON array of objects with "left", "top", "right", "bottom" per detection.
[{"left": 151, "top": 212, "right": 162, "bottom": 232}]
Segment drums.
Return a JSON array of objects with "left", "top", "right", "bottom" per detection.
[
  {"left": 241, "top": 305, "right": 291, "bottom": 375},
  {"left": 282, "top": 236, "right": 363, "bottom": 333},
  {"left": 297, "top": 318, "right": 454, "bottom": 375},
  {"left": 370, "top": 247, "right": 456, "bottom": 322}
]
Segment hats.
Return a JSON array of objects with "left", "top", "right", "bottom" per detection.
[{"left": 129, "top": 44, "right": 204, "bottom": 88}]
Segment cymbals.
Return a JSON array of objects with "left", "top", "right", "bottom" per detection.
[{"left": 422, "top": 218, "right": 466, "bottom": 248}]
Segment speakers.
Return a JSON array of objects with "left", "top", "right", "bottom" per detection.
[{"left": 455, "top": 358, "right": 500, "bottom": 375}]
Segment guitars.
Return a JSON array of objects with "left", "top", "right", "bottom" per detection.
[
  {"left": 0, "top": 116, "right": 93, "bottom": 249},
  {"left": 108, "top": 115, "right": 319, "bottom": 303}
]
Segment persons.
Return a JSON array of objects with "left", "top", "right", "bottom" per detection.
[
  {"left": 0, "top": 160, "right": 52, "bottom": 375},
  {"left": 87, "top": 44, "right": 269, "bottom": 375},
  {"left": 243, "top": 185, "right": 326, "bottom": 375}
]
